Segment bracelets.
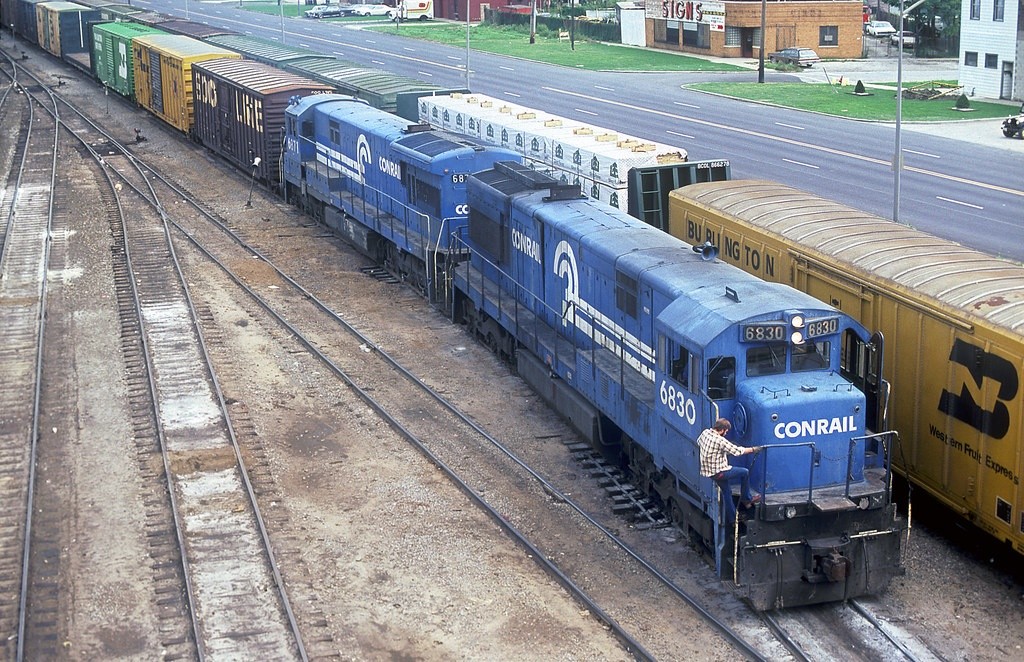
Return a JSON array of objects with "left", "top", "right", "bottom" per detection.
[{"left": 752, "top": 447, "right": 755, "bottom": 453}]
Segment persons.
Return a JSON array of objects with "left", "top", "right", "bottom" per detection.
[{"left": 696, "top": 418, "right": 760, "bottom": 537}]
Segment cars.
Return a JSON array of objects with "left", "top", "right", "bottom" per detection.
[
  {"left": 863, "top": 6, "right": 897, "bottom": 38},
  {"left": 303, "top": 1, "right": 396, "bottom": 19},
  {"left": 890, "top": 30, "right": 921, "bottom": 47},
  {"left": 768, "top": 46, "right": 822, "bottom": 67},
  {"left": 1000, "top": 102, "right": 1024, "bottom": 139}
]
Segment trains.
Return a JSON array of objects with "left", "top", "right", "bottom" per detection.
[{"left": 1, "top": 0, "right": 912, "bottom": 610}]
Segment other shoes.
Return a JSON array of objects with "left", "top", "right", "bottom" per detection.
[{"left": 746, "top": 494, "right": 761, "bottom": 508}]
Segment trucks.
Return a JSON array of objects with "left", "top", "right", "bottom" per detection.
[{"left": 388, "top": 0, "right": 435, "bottom": 23}]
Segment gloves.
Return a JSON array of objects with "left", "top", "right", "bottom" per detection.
[{"left": 753, "top": 446, "right": 760, "bottom": 452}]
